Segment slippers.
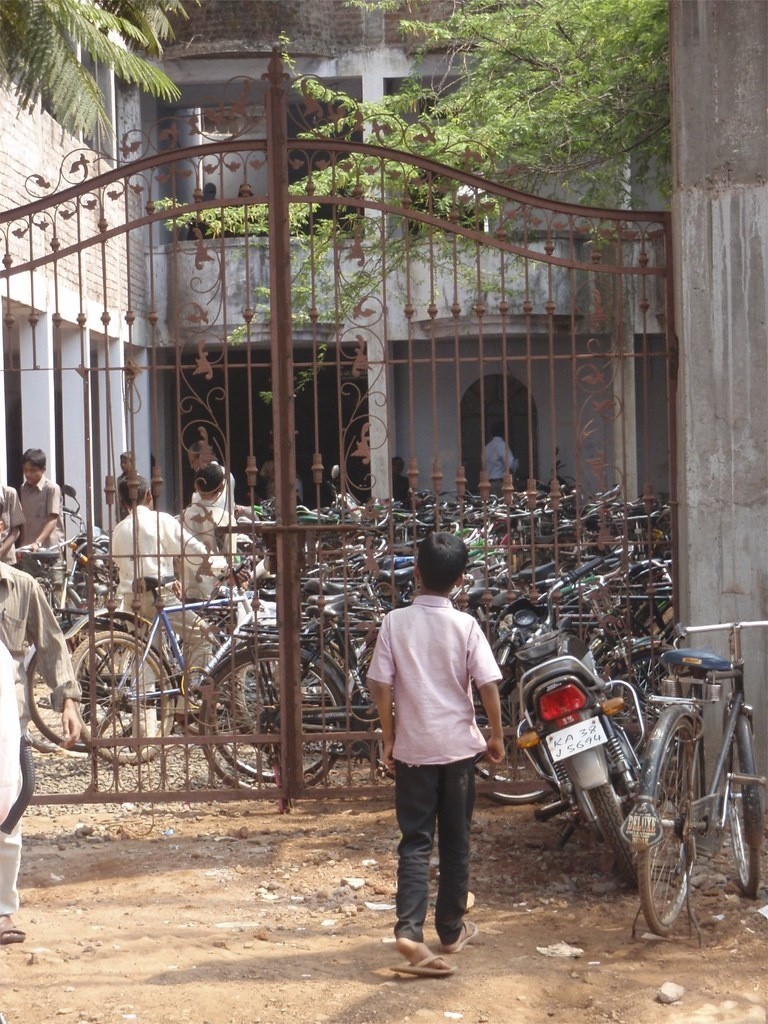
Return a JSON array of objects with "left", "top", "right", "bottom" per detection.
[
  {"left": 390, "top": 955, "right": 455, "bottom": 976},
  {"left": 448, "top": 921, "right": 478, "bottom": 954}
]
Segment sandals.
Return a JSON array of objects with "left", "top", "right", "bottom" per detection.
[{"left": 0, "top": 915, "right": 26, "bottom": 943}]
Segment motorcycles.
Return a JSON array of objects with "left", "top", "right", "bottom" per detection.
[{"left": 487, "top": 556, "right": 650, "bottom": 893}]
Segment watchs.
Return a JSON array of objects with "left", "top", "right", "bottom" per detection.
[{"left": 35, "top": 540, "right": 43, "bottom": 547}]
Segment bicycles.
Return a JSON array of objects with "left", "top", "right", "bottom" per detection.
[
  {"left": 15, "top": 446, "right": 683, "bottom": 808},
  {"left": 631, "top": 622, "right": 767, "bottom": 949}
]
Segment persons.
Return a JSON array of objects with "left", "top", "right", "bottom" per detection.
[
  {"left": 392, "top": 456, "right": 409, "bottom": 505},
  {"left": 189, "top": 441, "right": 237, "bottom": 514},
  {"left": 0, "top": 482, "right": 26, "bottom": 568},
  {"left": 115, "top": 452, "right": 139, "bottom": 520},
  {"left": 19, "top": 448, "right": 63, "bottom": 571},
  {"left": 112, "top": 475, "right": 251, "bottom": 763},
  {"left": 178, "top": 465, "right": 238, "bottom": 653},
  {"left": 235, "top": 183, "right": 266, "bottom": 236},
  {"left": 482, "top": 419, "right": 519, "bottom": 498},
  {"left": 187, "top": 183, "right": 221, "bottom": 240},
  {"left": 365, "top": 533, "right": 505, "bottom": 973},
  {"left": 0, "top": 504, "right": 84, "bottom": 942}
]
